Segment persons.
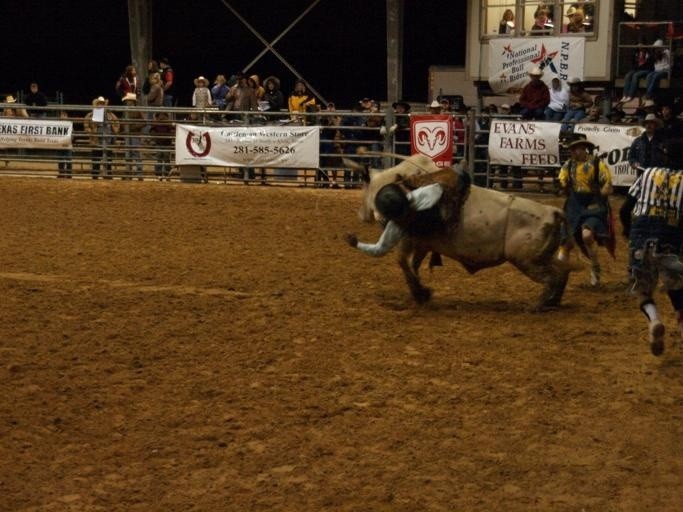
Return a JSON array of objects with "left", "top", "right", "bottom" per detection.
[
  {"left": 638, "top": 38, "right": 674, "bottom": 108},
  {"left": 53, "top": 111, "right": 73, "bottom": 179},
  {"left": 2, "top": 95, "right": 30, "bottom": 118},
  {"left": 556, "top": 133, "right": 617, "bottom": 289},
  {"left": 305, "top": 63, "right": 682, "bottom": 191},
  {"left": 82, "top": 58, "right": 319, "bottom": 183},
  {"left": 618, "top": 32, "right": 656, "bottom": 103},
  {"left": 22, "top": 82, "right": 49, "bottom": 118},
  {"left": 338, "top": 161, "right": 471, "bottom": 259},
  {"left": 523, "top": 0, "right": 593, "bottom": 34},
  {"left": 498, "top": 9, "right": 514, "bottom": 34},
  {"left": 617, "top": 140, "right": 683, "bottom": 356}
]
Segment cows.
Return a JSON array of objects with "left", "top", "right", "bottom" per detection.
[{"left": 341, "top": 153, "right": 593, "bottom": 315}]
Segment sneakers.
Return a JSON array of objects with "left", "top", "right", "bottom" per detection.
[
  {"left": 649, "top": 319, "right": 665, "bottom": 355},
  {"left": 591, "top": 265, "right": 600, "bottom": 285},
  {"left": 620, "top": 94, "right": 633, "bottom": 103}
]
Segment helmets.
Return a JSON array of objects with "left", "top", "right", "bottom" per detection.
[{"left": 376, "top": 183, "right": 409, "bottom": 222}]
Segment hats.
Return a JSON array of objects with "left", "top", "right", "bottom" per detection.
[
  {"left": 92, "top": 96, "right": 109, "bottom": 107},
  {"left": 426, "top": 98, "right": 449, "bottom": 108},
  {"left": 562, "top": 136, "right": 594, "bottom": 149},
  {"left": 527, "top": 65, "right": 544, "bottom": 75},
  {"left": 194, "top": 76, "right": 209, "bottom": 87},
  {"left": 566, "top": 6, "right": 576, "bottom": 17},
  {"left": 639, "top": 113, "right": 664, "bottom": 129},
  {"left": 3, "top": 96, "right": 17, "bottom": 103},
  {"left": 644, "top": 100, "right": 655, "bottom": 107},
  {"left": 567, "top": 78, "right": 581, "bottom": 84},
  {"left": 122, "top": 92, "right": 137, "bottom": 101},
  {"left": 392, "top": 100, "right": 410, "bottom": 111}
]
